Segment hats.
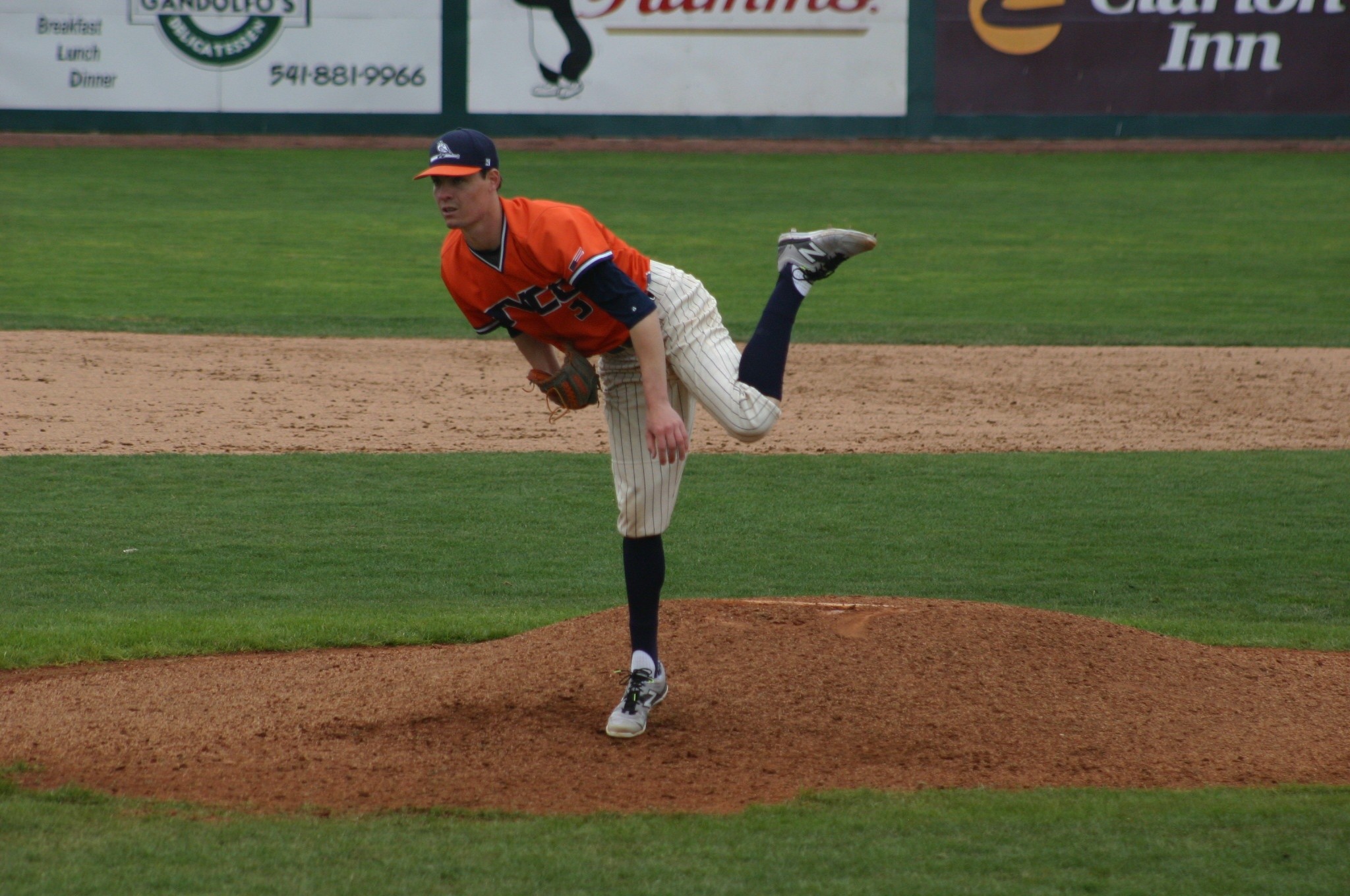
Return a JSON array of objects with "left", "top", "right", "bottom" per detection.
[{"left": 414, "top": 128, "right": 500, "bottom": 179}]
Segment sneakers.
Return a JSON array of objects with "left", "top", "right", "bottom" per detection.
[
  {"left": 777, "top": 228, "right": 877, "bottom": 296},
  {"left": 606, "top": 650, "right": 668, "bottom": 737}
]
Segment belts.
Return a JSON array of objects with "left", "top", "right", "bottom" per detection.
[{"left": 607, "top": 291, "right": 656, "bottom": 356}]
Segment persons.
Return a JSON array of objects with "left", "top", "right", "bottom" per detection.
[{"left": 415, "top": 128, "right": 877, "bottom": 738}]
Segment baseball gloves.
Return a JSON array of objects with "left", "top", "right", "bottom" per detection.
[{"left": 525, "top": 344, "right": 600, "bottom": 410}]
